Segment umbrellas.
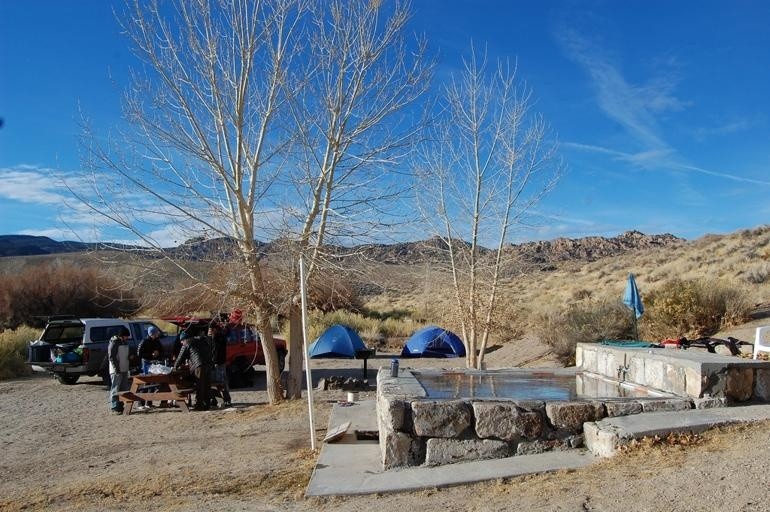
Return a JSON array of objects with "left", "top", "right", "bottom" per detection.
[{"left": 622, "top": 272, "right": 644, "bottom": 319}]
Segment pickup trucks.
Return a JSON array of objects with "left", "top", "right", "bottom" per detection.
[{"left": 19, "top": 312, "right": 179, "bottom": 386}]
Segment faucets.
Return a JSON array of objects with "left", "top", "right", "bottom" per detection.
[{"left": 617, "top": 364, "right": 630, "bottom": 379}]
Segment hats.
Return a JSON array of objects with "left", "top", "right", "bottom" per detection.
[
  {"left": 180, "top": 333, "right": 189, "bottom": 341},
  {"left": 119, "top": 328, "right": 131, "bottom": 337},
  {"left": 148, "top": 326, "right": 158, "bottom": 336}
]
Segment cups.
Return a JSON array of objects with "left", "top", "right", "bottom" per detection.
[{"left": 347, "top": 392, "right": 355, "bottom": 403}]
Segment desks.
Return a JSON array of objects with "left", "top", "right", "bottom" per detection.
[{"left": 123, "top": 375, "right": 191, "bottom": 415}]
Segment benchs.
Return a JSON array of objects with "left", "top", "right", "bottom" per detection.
[
  {"left": 114, "top": 384, "right": 160, "bottom": 415},
  {"left": 177, "top": 387, "right": 193, "bottom": 412}
]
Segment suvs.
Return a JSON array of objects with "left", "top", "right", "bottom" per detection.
[{"left": 154, "top": 314, "right": 280, "bottom": 389}]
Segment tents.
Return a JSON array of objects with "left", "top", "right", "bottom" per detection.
[
  {"left": 400, "top": 327, "right": 465, "bottom": 359},
  {"left": 310, "top": 324, "right": 365, "bottom": 358}
]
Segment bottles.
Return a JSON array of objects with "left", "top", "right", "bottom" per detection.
[{"left": 390, "top": 359, "right": 399, "bottom": 377}]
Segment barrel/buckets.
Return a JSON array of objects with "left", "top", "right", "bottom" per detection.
[{"left": 347, "top": 392, "right": 359, "bottom": 402}]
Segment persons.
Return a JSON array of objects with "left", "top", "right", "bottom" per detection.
[
  {"left": 106, "top": 327, "right": 130, "bottom": 412},
  {"left": 137, "top": 323, "right": 231, "bottom": 411}
]
